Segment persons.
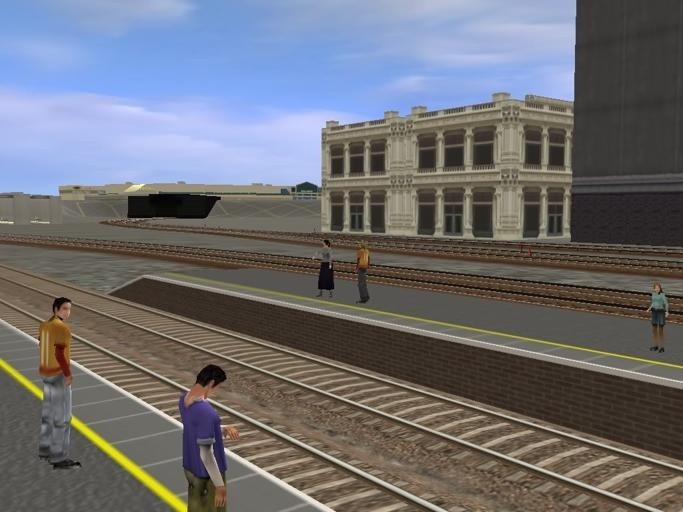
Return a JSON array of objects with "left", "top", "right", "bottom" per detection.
[
  {"left": 35, "top": 295, "right": 82, "bottom": 471},
  {"left": 353, "top": 243, "right": 370, "bottom": 304},
  {"left": 177, "top": 363, "right": 240, "bottom": 512},
  {"left": 311, "top": 238, "right": 335, "bottom": 299},
  {"left": 644, "top": 282, "right": 669, "bottom": 353}
]
